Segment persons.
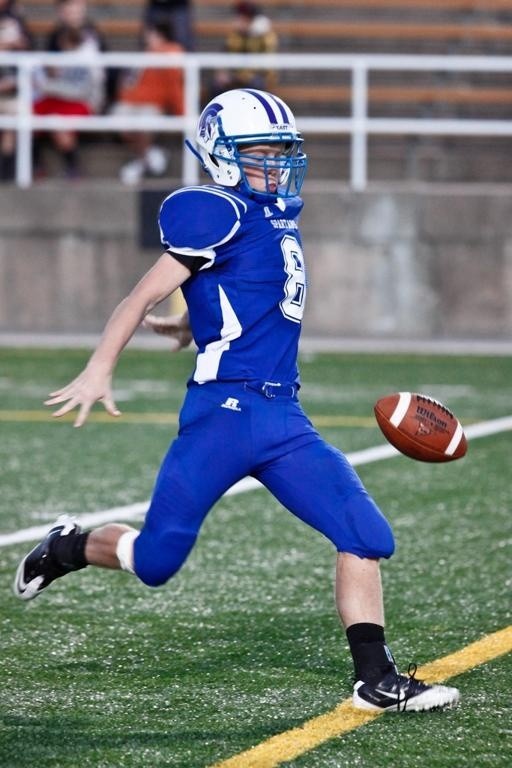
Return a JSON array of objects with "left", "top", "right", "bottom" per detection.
[
  {"left": 140, "top": 0, "right": 193, "bottom": 54},
  {"left": 107, "top": 16, "right": 206, "bottom": 182},
  {"left": 206, "top": 0, "right": 278, "bottom": 102},
  {"left": 44, "top": 1, "right": 119, "bottom": 104},
  {"left": 0, "top": 0, "right": 34, "bottom": 51},
  {"left": 1, "top": 66, "right": 20, "bottom": 182},
  {"left": 9, "top": 86, "right": 462, "bottom": 717},
  {"left": 30, "top": 21, "right": 108, "bottom": 178}
]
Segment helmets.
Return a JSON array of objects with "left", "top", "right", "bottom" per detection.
[{"left": 185, "top": 89, "right": 309, "bottom": 203}]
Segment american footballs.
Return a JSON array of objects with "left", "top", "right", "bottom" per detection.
[{"left": 374, "top": 392, "right": 467, "bottom": 463}]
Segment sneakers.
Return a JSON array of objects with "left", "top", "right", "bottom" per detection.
[
  {"left": 352, "top": 675, "right": 462, "bottom": 714},
  {"left": 12, "top": 516, "right": 80, "bottom": 601}
]
